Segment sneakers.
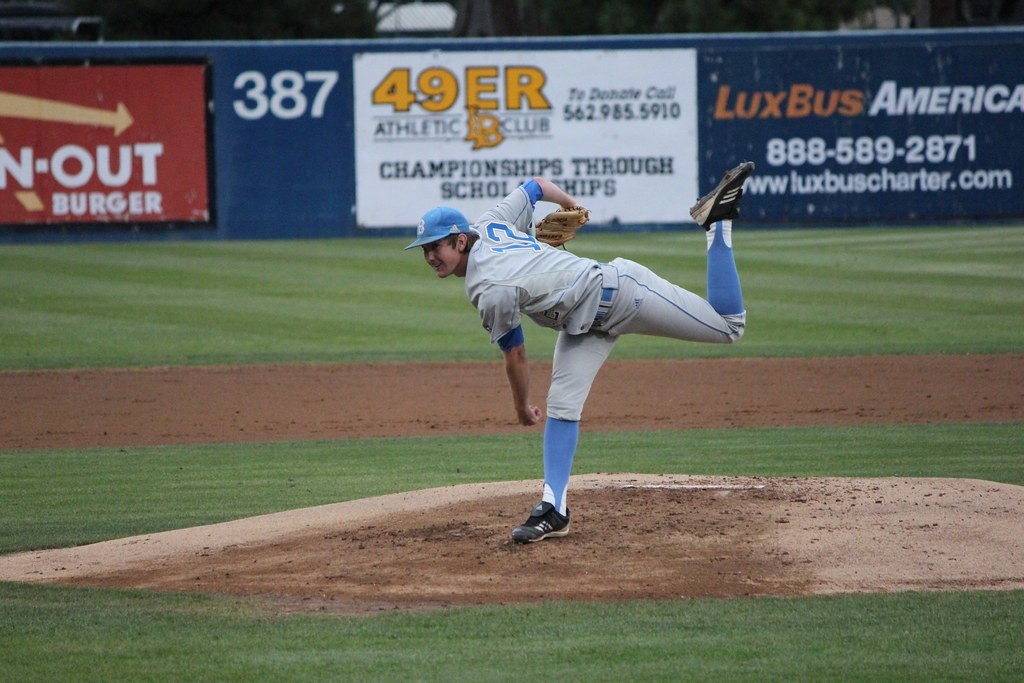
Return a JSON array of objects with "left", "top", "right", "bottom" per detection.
[
  {"left": 690, "top": 162, "right": 755, "bottom": 231},
  {"left": 512, "top": 501, "right": 571, "bottom": 541}
]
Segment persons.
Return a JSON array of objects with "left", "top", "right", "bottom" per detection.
[{"left": 405, "top": 162, "right": 755, "bottom": 542}]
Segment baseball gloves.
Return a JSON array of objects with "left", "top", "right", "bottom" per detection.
[{"left": 534, "top": 206, "right": 593, "bottom": 251}]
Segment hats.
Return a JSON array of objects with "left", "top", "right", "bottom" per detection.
[{"left": 405, "top": 207, "right": 470, "bottom": 250}]
